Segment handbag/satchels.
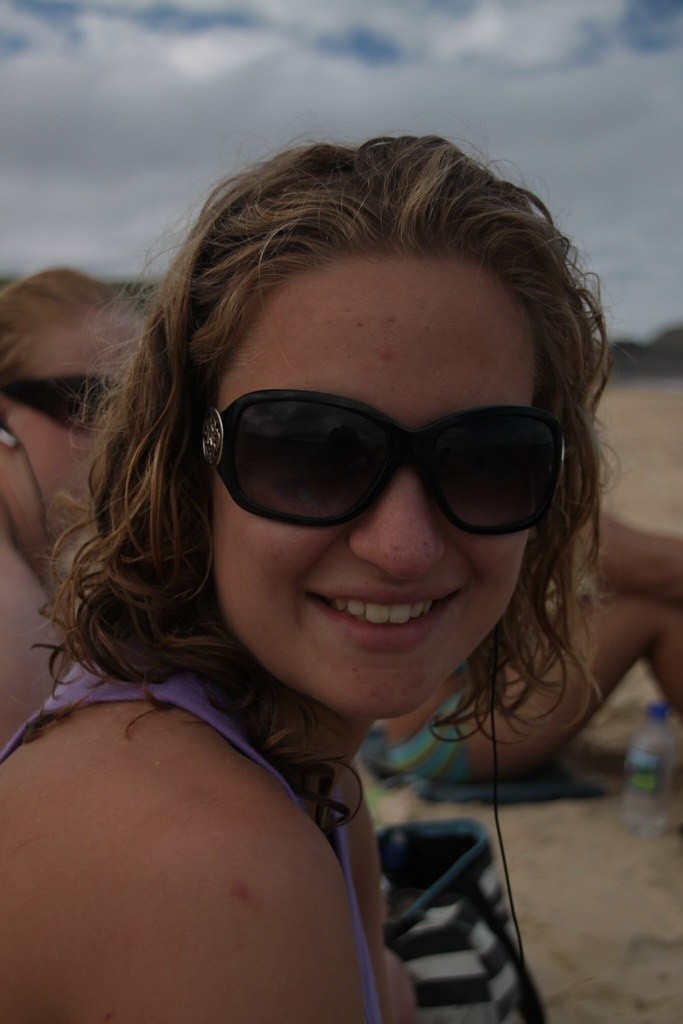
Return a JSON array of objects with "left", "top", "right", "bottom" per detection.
[{"left": 371, "top": 819, "right": 546, "bottom": 1024}]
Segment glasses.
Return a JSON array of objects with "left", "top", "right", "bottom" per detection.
[
  {"left": 199, "top": 388, "right": 568, "bottom": 537},
  {"left": 0, "top": 371, "right": 123, "bottom": 430}
]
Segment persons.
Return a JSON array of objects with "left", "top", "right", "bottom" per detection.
[
  {"left": 361, "top": 506, "right": 683, "bottom": 784},
  {"left": 0, "top": 135, "right": 619, "bottom": 1024}
]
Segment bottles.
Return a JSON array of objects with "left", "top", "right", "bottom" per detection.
[{"left": 619, "top": 704, "right": 677, "bottom": 838}]
